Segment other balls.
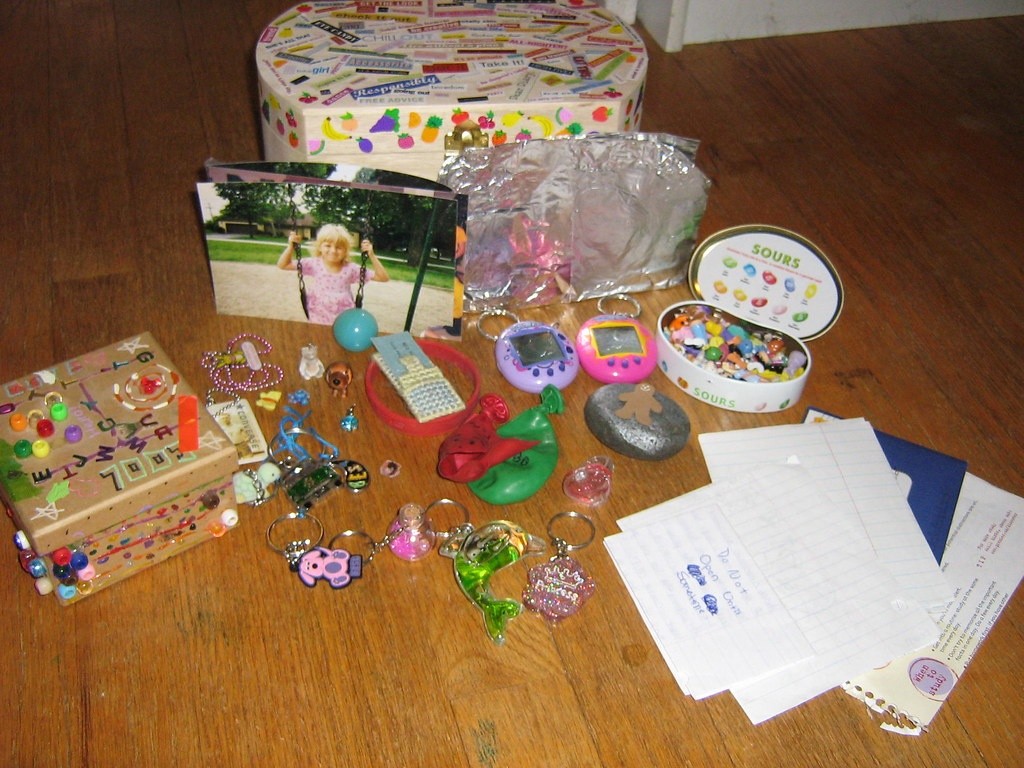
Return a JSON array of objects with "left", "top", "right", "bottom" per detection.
[{"left": 332, "top": 307, "right": 379, "bottom": 352}]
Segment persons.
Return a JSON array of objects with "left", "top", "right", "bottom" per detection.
[{"left": 275, "top": 222, "right": 391, "bottom": 326}]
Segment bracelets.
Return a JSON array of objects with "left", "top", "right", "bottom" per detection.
[{"left": 365, "top": 342, "right": 482, "bottom": 436}]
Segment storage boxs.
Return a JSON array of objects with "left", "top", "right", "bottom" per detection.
[
  {"left": 652, "top": 222, "right": 846, "bottom": 413},
  {"left": 0, "top": 330, "right": 239, "bottom": 606},
  {"left": 254, "top": 1, "right": 652, "bottom": 182}
]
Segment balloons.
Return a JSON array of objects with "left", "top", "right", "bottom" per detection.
[{"left": 436, "top": 385, "right": 564, "bottom": 507}]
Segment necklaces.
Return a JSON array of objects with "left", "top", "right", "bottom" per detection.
[{"left": 202, "top": 333, "right": 283, "bottom": 390}]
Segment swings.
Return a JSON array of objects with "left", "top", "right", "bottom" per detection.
[{"left": 287, "top": 183, "right": 374, "bottom": 321}]
